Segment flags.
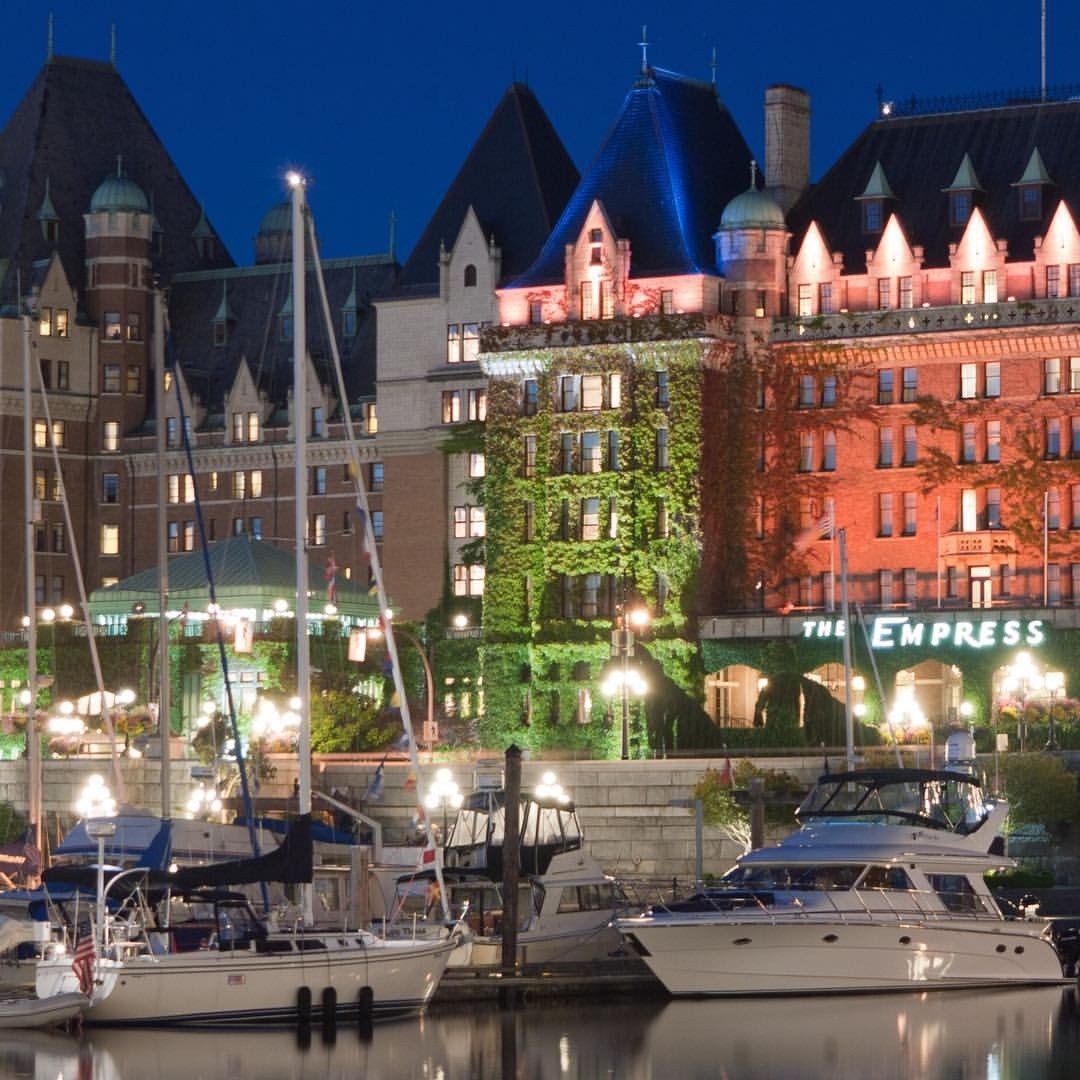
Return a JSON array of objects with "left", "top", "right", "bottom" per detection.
[
  {"left": 24, "top": 828, "right": 40, "bottom": 874},
  {"left": 324, "top": 558, "right": 337, "bottom": 600},
  {"left": 363, "top": 533, "right": 408, "bottom": 749},
  {"left": 407, "top": 771, "right": 416, "bottom": 791},
  {"left": 71, "top": 920, "right": 95, "bottom": 998},
  {"left": 410, "top": 808, "right": 423, "bottom": 828},
  {"left": 427, "top": 881, "right": 440, "bottom": 899},
  {"left": 423, "top": 850, "right": 435, "bottom": 864},
  {"left": 367, "top": 765, "right": 385, "bottom": 801}
]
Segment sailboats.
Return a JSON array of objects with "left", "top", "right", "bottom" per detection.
[{"left": 0, "top": 171, "right": 659, "bottom": 1030}]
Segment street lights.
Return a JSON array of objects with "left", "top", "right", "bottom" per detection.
[{"left": 621, "top": 563, "right": 648, "bottom": 758}]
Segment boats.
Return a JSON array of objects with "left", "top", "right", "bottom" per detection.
[{"left": 607, "top": 526, "right": 1080, "bottom": 1001}]
